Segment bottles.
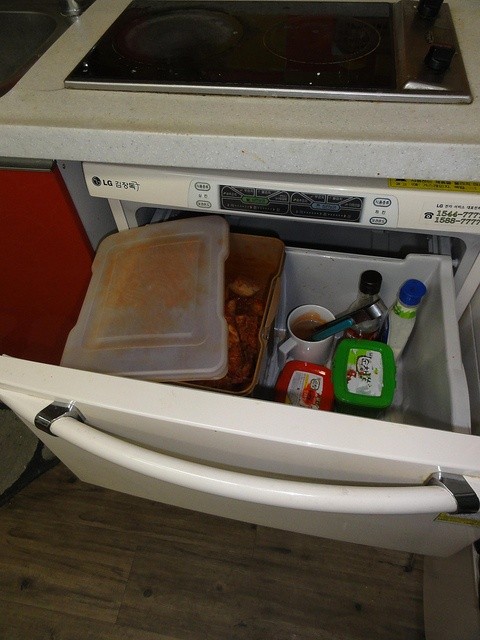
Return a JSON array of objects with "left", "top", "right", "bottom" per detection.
[
  {"left": 379, "top": 279, "right": 426, "bottom": 361},
  {"left": 343, "top": 269, "right": 390, "bottom": 341}
]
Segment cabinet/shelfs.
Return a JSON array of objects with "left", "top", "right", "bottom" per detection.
[{"left": 0, "top": 207, "right": 479, "bottom": 559}]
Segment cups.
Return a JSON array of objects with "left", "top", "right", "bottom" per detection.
[{"left": 277, "top": 303, "right": 337, "bottom": 370}]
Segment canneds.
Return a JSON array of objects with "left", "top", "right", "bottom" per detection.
[
  {"left": 274, "top": 361, "right": 333, "bottom": 411},
  {"left": 331, "top": 338, "right": 396, "bottom": 419}
]
